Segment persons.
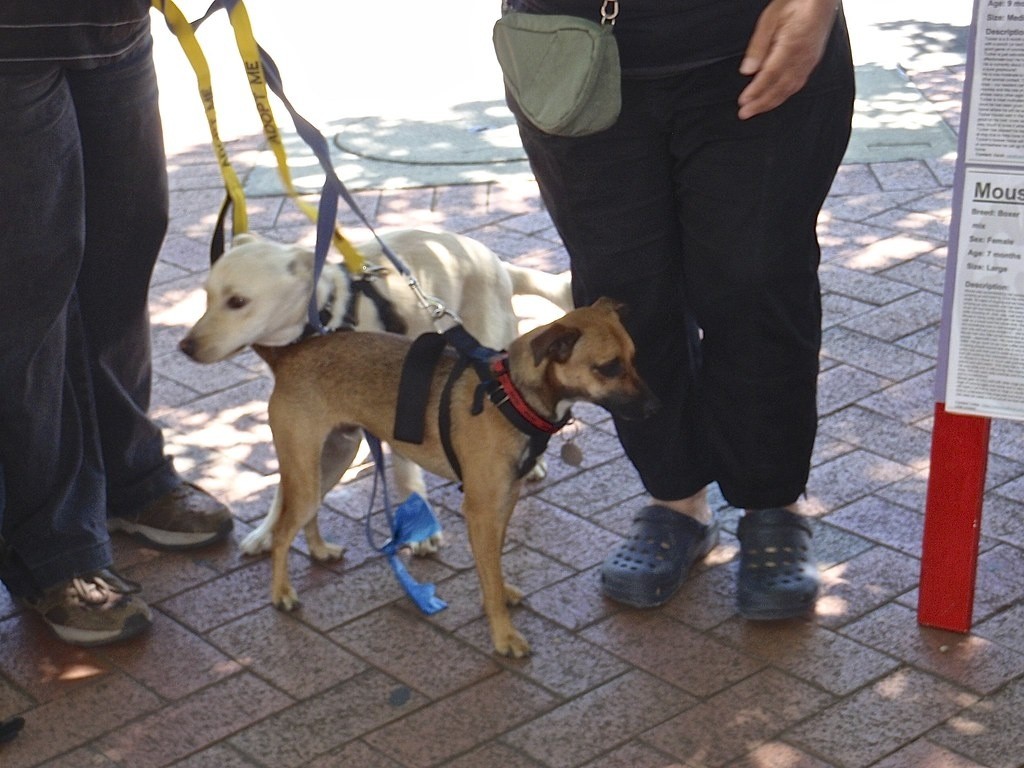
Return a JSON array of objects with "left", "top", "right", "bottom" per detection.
[
  {"left": 0, "top": 0, "right": 232, "bottom": 646},
  {"left": 499, "top": 0, "right": 854, "bottom": 622}
]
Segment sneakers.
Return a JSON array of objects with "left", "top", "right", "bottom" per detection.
[
  {"left": 11, "top": 565, "right": 155, "bottom": 646},
  {"left": 106, "top": 455, "right": 234, "bottom": 553}
]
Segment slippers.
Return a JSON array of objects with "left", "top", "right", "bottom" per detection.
[
  {"left": 598, "top": 503, "right": 721, "bottom": 608},
  {"left": 735, "top": 507, "right": 820, "bottom": 620}
]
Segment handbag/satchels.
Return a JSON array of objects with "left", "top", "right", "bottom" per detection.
[{"left": 492, "top": 14, "right": 622, "bottom": 139}]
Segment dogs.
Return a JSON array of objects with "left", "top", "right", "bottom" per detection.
[
  {"left": 178, "top": 229, "right": 578, "bottom": 560},
  {"left": 267, "top": 296, "right": 661, "bottom": 659}
]
